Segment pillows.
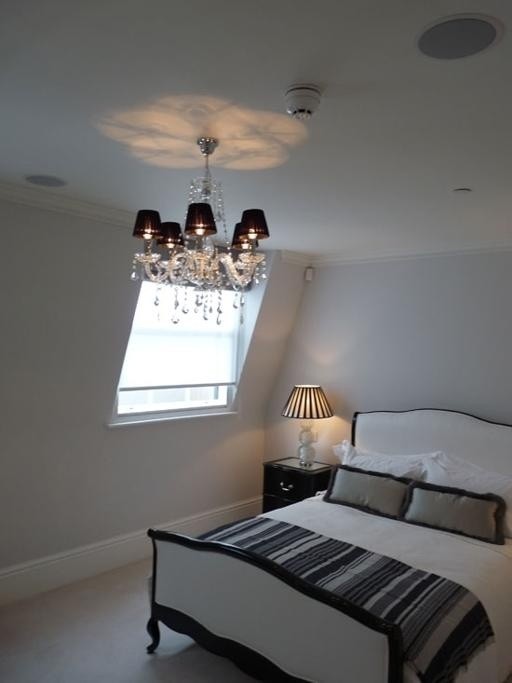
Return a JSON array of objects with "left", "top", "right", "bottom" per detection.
[{"left": 323, "top": 438, "right": 512, "bottom": 544}]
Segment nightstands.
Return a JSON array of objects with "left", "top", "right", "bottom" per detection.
[{"left": 262, "top": 456, "right": 332, "bottom": 513}]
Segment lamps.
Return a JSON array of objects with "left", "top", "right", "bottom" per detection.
[
  {"left": 130, "top": 137, "right": 272, "bottom": 327},
  {"left": 281, "top": 384, "right": 335, "bottom": 468}
]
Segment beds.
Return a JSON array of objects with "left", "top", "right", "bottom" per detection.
[{"left": 146, "top": 408, "right": 511, "bottom": 682}]
